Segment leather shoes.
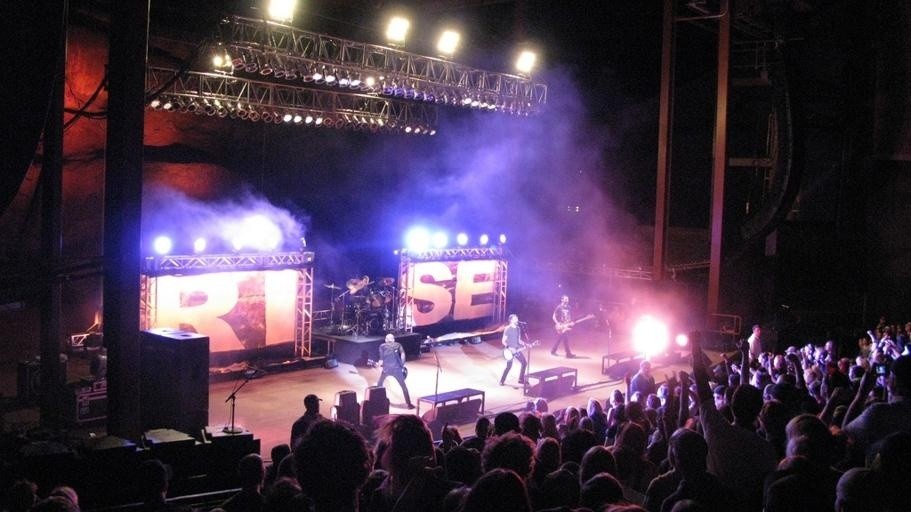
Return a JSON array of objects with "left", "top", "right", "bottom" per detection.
[
  {"left": 519, "top": 379, "right": 530, "bottom": 384},
  {"left": 567, "top": 353, "right": 576, "bottom": 357},
  {"left": 498, "top": 380, "right": 504, "bottom": 385},
  {"left": 552, "top": 351, "right": 559, "bottom": 356},
  {"left": 408, "top": 404, "right": 415, "bottom": 409}
]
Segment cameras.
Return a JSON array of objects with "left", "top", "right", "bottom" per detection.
[{"left": 875, "top": 364, "right": 890, "bottom": 376}]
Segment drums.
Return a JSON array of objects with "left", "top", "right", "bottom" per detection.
[
  {"left": 366, "top": 288, "right": 392, "bottom": 307},
  {"left": 355, "top": 309, "right": 382, "bottom": 332}
]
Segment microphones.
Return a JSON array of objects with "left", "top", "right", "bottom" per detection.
[
  {"left": 246, "top": 365, "right": 266, "bottom": 374},
  {"left": 517, "top": 320, "right": 528, "bottom": 325},
  {"left": 427, "top": 335, "right": 435, "bottom": 348}
]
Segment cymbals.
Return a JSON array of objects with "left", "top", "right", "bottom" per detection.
[
  {"left": 346, "top": 279, "right": 363, "bottom": 290},
  {"left": 378, "top": 278, "right": 395, "bottom": 287}
]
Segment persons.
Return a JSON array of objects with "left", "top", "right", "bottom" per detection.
[
  {"left": 135, "top": 459, "right": 183, "bottom": 507},
  {"left": 550, "top": 297, "right": 576, "bottom": 358},
  {"left": 64, "top": 333, "right": 107, "bottom": 389},
  {"left": 630, "top": 360, "right": 656, "bottom": 396},
  {"left": 4, "top": 478, "right": 80, "bottom": 508},
  {"left": 435, "top": 371, "right": 731, "bottom": 509},
  {"left": 211, "top": 391, "right": 453, "bottom": 509},
  {"left": 500, "top": 315, "right": 529, "bottom": 385},
  {"left": 377, "top": 333, "right": 415, "bottom": 410},
  {"left": 688, "top": 316, "right": 909, "bottom": 509},
  {"left": 747, "top": 325, "right": 762, "bottom": 364}
]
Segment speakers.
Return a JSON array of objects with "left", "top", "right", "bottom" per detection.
[
  {"left": 737, "top": 72, "right": 805, "bottom": 242},
  {"left": 606, "top": 358, "right": 643, "bottom": 377},
  {"left": 423, "top": 398, "right": 481, "bottom": 425},
  {"left": 0, "top": 0, "right": 68, "bottom": 216},
  {"left": 141, "top": 326, "right": 208, "bottom": 430},
  {"left": 527, "top": 375, "right": 575, "bottom": 398}
]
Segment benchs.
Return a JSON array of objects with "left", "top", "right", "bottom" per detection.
[
  {"left": 414, "top": 388, "right": 486, "bottom": 430},
  {"left": 525, "top": 365, "right": 581, "bottom": 400},
  {"left": 601, "top": 352, "right": 648, "bottom": 377}
]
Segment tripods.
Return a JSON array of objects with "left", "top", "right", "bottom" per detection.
[{"left": 327, "top": 291, "right": 400, "bottom": 336}]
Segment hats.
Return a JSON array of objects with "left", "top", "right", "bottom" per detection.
[{"left": 305, "top": 395, "right": 323, "bottom": 402}]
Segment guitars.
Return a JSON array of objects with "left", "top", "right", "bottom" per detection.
[
  {"left": 504, "top": 340, "right": 540, "bottom": 360},
  {"left": 367, "top": 359, "right": 407, "bottom": 379},
  {"left": 555, "top": 314, "right": 595, "bottom": 335}
]
